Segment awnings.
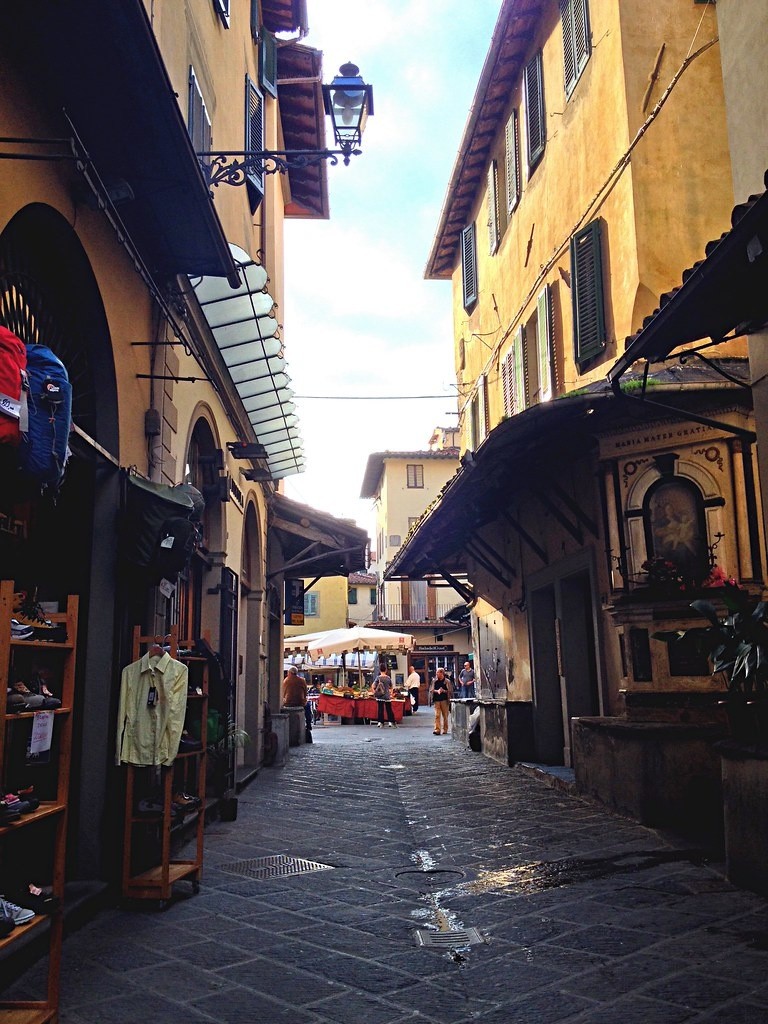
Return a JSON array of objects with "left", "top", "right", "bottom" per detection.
[
  {"left": 606, "top": 169, "right": 768, "bottom": 388},
  {"left": 382, "top": 359, "right": 751, "bottom": 579},
  {"left": 268, "top": 490, "right": 372, "bottom": 578},
  {"left": 284, "top": 650, "right": 378, "bottom": 671}
]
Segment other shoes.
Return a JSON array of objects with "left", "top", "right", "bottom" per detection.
[
  {"left": 0, "top": 785, "right": 39, "bottom": 822},
  {"left": 188, "top": 685, "right": 197, "bottom": 695},
  {"left": 306, "top": 725, "right": 312, "bottom": 730},
  {"left": 378, "top": 722, "right": 384, "bottom": 728},
  {"left": 179, "top": 731, "right": 201, "bottom": 752},
  {"left": 162, "top": 644, "right": 191, "bottom": 657},
  {"left": 433, "top": 729, "right": 440, "bottom": 735},
  {"left": 139, "top": 798, "right": 184, "bottom": 816},
  {"left": 173, "top": 792, "right": 199, "bottom": 808},
  {"left": 388, "top": 722, "right": 393, "bottom": 727},
  {"left": 443, "top": 729, "right": 447, "bottom": 734}
]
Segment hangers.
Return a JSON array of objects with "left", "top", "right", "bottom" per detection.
[{"left": 123, "top": 634, "right": 187, "bottom": 675}]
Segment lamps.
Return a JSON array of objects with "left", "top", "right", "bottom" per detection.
[
  {"left": 226, "top": 441, "right": 270, "bottom": 460},
  {"left": 441, "top": 605, "right": 472, "bottom": 627},
  {"left": 240, "top": 467, "right": 272, "bottom": 485},
  {"left": 194, "top": 59, "right": 374, "bottom": 194}
]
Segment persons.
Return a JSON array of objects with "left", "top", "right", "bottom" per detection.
[
  {"left": 430, "top": 667, "right": 453, "bottom": 735},
  {"left": 404, "top": 666, "right": 420, "bottom": 713},
  {"left": 372, "top": 666, "right": 397, "bottom": 728},
  {"left": 458, "top": 661, "right": 476, "bottom": 698},
  {"left": 282, "top": 666, "right": 312, "bottom": 728}
]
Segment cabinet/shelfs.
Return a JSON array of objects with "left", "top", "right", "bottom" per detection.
[
  {"left": 123, "top": 625, "right": 210, "bottom": 910},
  {"left": 0, "top": 579, "right": 80, "bottom": 1024},
  {"left": 320, "top": 693, "right": 406, "bottom": 726}
]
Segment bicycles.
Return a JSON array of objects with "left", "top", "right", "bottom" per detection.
[{"left": 309, "top": 685, "right": 319, "bottom": 725}]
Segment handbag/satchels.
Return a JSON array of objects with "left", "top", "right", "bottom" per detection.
[{"left": 374, "top": 676, "right": 385, "bottom": 697}]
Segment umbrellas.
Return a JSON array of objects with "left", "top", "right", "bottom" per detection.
[{"left": 284, "top": 625, "right": 417, "bottom": 696}]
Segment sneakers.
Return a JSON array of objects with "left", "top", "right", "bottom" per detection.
[
  {"left": 27, "top": 678, "right": 61, "bottom": 710},
  {"left": 0, "top": 913, "right": 16, "bottom": 937},
  {"left": 4, "top": 883, "right": 59, "bottom": 915},
  {"left": 0, "top": 895, "right": 35, "bottom": 925},
  {"left": 13, "top": 682, "right": 46, "bottom": 709},
  {"left": 12, "top": 587, "right": 64, "bottom": 636},
  {"left": 10, "top": 620, "right": 33, "bottom": 639},
  {"left": 6, "top": 688, "right": 26, "bottom": 713}
]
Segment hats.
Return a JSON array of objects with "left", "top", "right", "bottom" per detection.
[{"left": 464, "top": 662, "right": 470, "bottom": 665}]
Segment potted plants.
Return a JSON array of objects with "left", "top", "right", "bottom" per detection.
[
  {"left": 210, "top": 713, "right": 253, "bottom": 819},
  {"left": 636, "top": 551, "right": 768, "bottom": 897}
]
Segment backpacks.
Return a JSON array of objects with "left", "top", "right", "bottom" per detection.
[
  {"left": 25, "top": 343, "right": 72, "bottom": 494},
  {"left": 0, "top": 325, "right": 29, "bottom": 448}
]
